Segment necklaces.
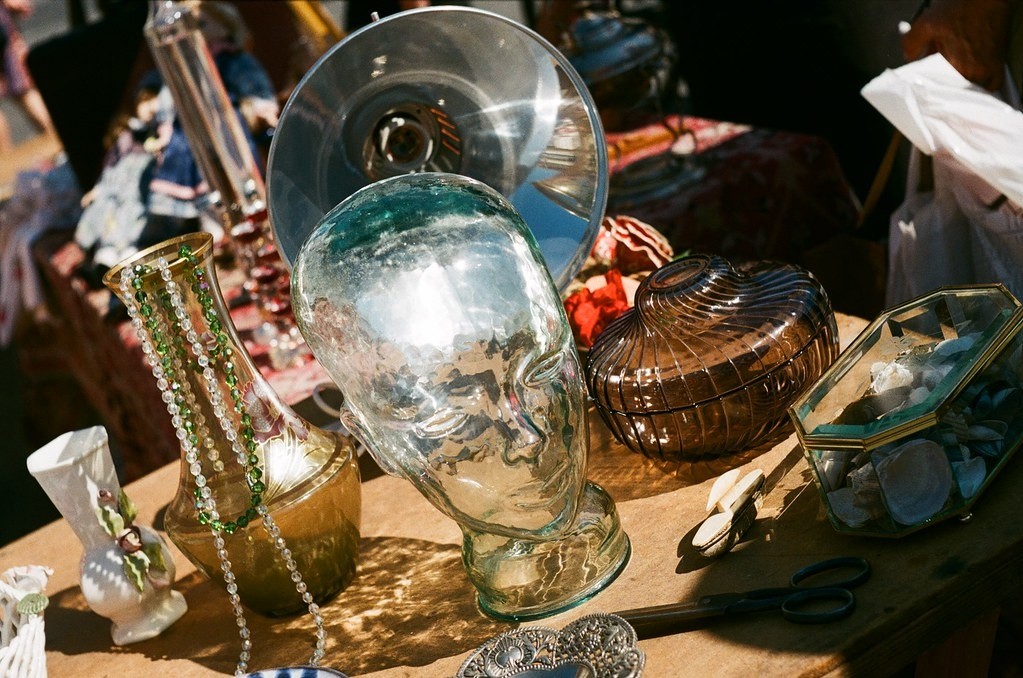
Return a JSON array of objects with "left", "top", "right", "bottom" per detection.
[{"left": 121, "top": 246, "right": 328, "bottom": 678}]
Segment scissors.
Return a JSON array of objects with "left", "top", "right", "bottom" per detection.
[{"left": 610, "top": 554, "right": 874, "bottom": 628}]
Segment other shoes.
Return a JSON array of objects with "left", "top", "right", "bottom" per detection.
[
  {"left": 74, "top": 261, "right": 109, "bottom": 294},
  {"left": 100, "top": 304, "right": 130, "bottom": 326}
]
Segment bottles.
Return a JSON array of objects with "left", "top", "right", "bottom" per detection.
[
  {"left": 100, "top": 232, "right": 365, "bottom": 616},
  {"left": 27, "top": 424, "right": 190, "bottom": 646}
]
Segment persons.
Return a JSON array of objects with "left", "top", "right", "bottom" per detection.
[
  {"left": 289, "top": 172, "right": 632, "bottom": 623},
  {"left": 0, "top": 0, "right": 278, "bottom": 327}
]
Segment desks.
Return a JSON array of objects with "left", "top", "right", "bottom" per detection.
[{"left": 1, "top": 311, "right": 1023, "bottom": 678}]
